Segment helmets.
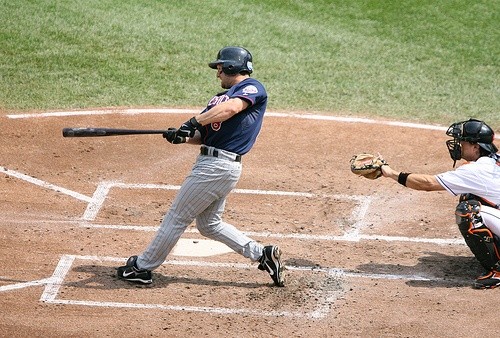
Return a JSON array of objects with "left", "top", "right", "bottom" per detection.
[
  {"left": 208, "top": 46, "right": 252, "bottom": 75},
  {"left": 460, "top": 121, "right": 497, "bottom": 153}
]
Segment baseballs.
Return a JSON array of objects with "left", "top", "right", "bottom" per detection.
[{"left": 62, "top": 126, "right": 195, "bottom": 138}]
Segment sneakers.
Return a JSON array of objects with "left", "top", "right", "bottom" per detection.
[
  {"left": 117, "top": 256, "right": 153, "bottom": 285},
  {"left": 258, "top": 246, "right": 285, "bottom": 286},
  {"left": 473, "top": 270, "right": 500, "bottom": 289}
]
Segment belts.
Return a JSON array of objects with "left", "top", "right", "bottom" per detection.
[{"left": 200, "top": 147, "right": 241, "bottom": 162}]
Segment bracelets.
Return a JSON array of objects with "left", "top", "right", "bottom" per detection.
[{"left": 398, "top": 172, "right": 411, "bottom": 187}]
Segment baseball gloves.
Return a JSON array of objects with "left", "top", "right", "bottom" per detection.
[{"left": 350, "top": 153, "right": 388, "bottom": 180}]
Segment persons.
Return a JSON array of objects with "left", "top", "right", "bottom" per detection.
[
  {"left": 117, "top": 46, "right": 286, "bottom": 286},
  {"left": 351, "top": 119, "right": 500, "bottom": 288}
]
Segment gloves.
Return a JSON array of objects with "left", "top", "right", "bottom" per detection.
[
  {"left": 178, "top": 117, "right": 200, "bottom": 137},
  {"left": 163, "top": 128, "right": 186, "bottom": 144}
]
